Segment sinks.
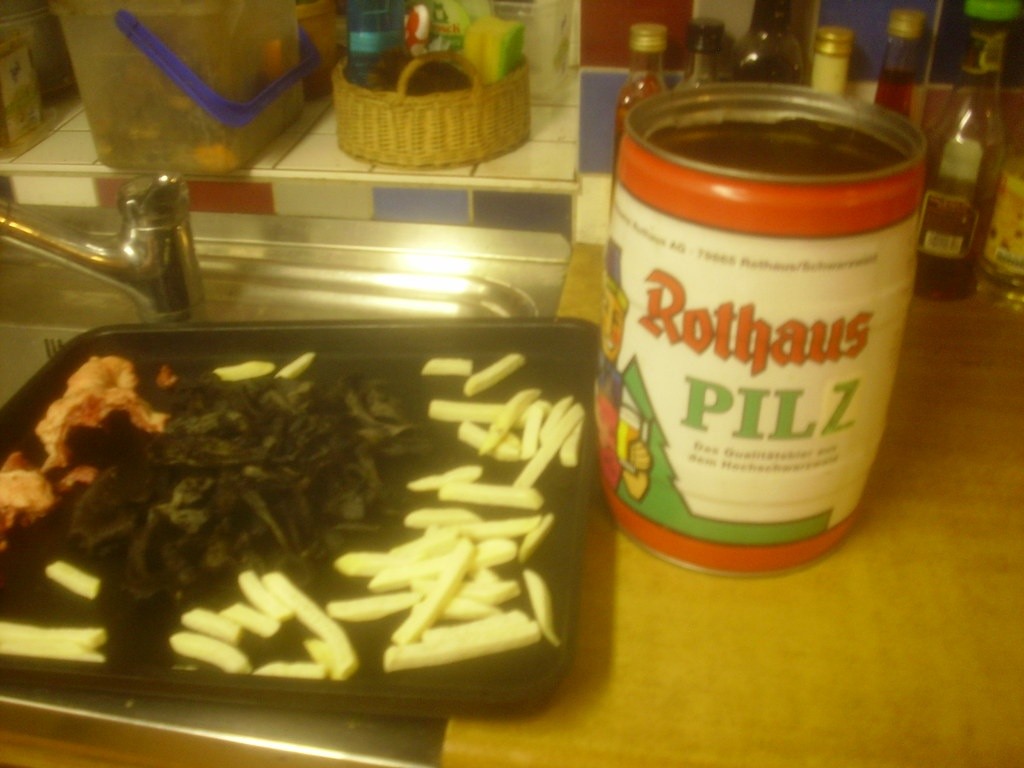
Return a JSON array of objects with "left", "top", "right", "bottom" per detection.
[{"left": 1, "top": 319, "right": 89, "bottom": 409}]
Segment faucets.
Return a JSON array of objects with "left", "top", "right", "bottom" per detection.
[{"left": 0, "top": 169, "right": 205, "bottom": 323}]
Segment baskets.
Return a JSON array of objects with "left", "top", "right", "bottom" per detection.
[{"left": 332, "top": 55, "right": 529, "bottom": 170}]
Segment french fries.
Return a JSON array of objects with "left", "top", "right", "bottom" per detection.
[{"left": 0, "top": 352, "right": 584, "bottom": 681}]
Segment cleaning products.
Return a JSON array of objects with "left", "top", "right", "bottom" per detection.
[{"left": 346, "top": 0, "right": 490, "bottom": 86}]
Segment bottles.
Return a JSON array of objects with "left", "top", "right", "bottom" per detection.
[
  {"left": 613, "top": 23, "right": 669, "bottom": 170},
  {"left": 972, "top": 152, "right": 1024, "bottom": 314},
  {"left": 673, "top": 17, "right": 726, "bottom": 88},
  {"left": 873, "top": 9, "right": 927, "bottom": 115},
  {"left": 734, "top": 0, "right": 803, "bottom": 83},
  {"left": 812, "top": 26, "right": 854, "bottom": 94},
  {"left": 916, "top": 1, "right": 1020, "bottom": 300},
  {"left": 298, "top": 0, "right": 404, "bottom": 99}
]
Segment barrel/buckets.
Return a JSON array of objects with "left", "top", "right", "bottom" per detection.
[{"left": 592, "top": 84, "right": 927, "bottom": 579}]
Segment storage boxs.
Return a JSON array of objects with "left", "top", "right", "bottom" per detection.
[{"left": 50, "top": 0, "right": 321, "bottom": 179}]
